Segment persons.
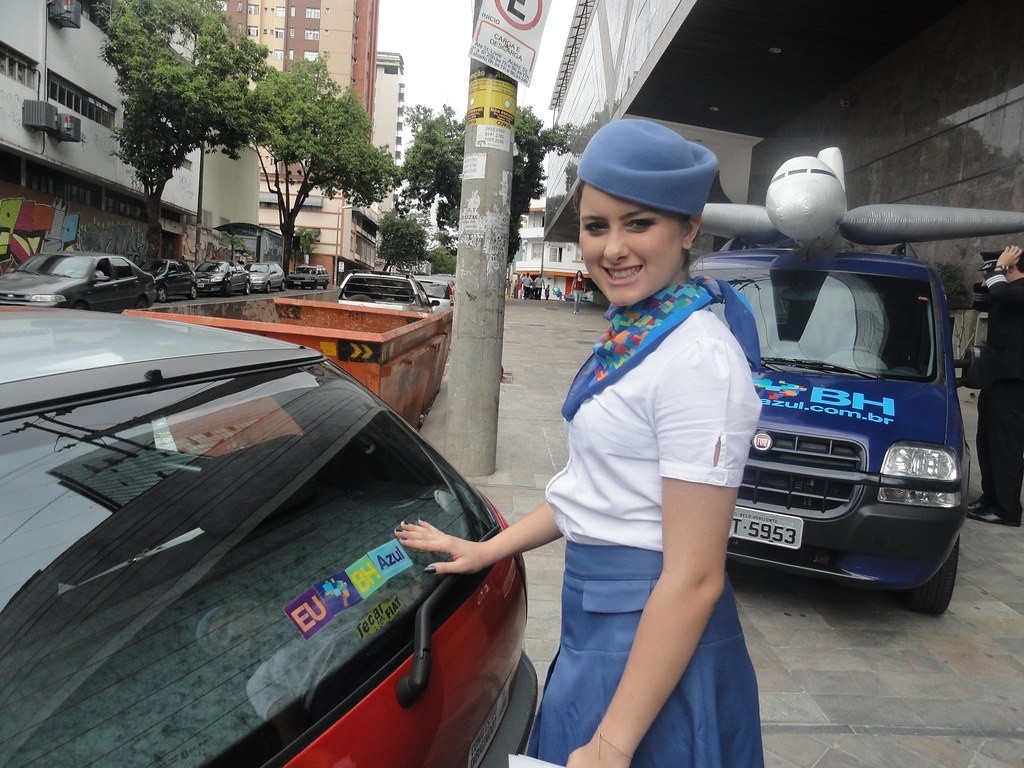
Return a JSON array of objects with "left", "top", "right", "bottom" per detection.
[
  {"left": 523, "top": 272, "right": 533, "bottom": 301},
  {"left": 545, "top": 284, "right": 550, "bottom": 300},
  {"left": 571, "top": 270, "right": 586, "bottom": 315},
  {"left": 534, "top": 274, "right": 545, "bottom": 301},
  {"left": 966, "top": 245, "right": 1024, "bottom": 528},
  {"left": 197, "top": 552, "right": 445, "bottom": 721},
  {"left": 395, "top": 119, "right": 766, "bottom": 768}
]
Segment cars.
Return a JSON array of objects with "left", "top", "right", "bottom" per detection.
[
  {"left": 1, "top": 306, "right": 536, "bottom": 767},
  {"left": 286, "top": 265, "right": 330, "bottom": 290},
  {"left": 565, "top": 291, "right": 587, "bottom": 302},
  {"left": 0, "top": 252, "right": 157, "bottom": 312},
  {"left": 135, "top": 259, "right": 197, "bottom": 302},
  {"left": 243, "top": 262, "right": 286, "bottom": 293},
  {"left": 414, "top": 276, "right": 455, "bottom": 314},
  {"left": 194, "top": 260, "right": 251, "bottom": 297},
  {"left": 584, "top": 291, "right": 593, "bottom": 302}
]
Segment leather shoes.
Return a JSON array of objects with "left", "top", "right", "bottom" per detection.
[{"left": 967, "top": 502, "right": 1020, "bottom": 527}]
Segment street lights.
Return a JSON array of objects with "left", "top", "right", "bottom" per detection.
[{"left": 333, "top": 206, "right": 353, "bottom": 289}]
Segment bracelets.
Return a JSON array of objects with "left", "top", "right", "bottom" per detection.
[{"left": 597, "top": 726, "right": 633, "bottom": 760}]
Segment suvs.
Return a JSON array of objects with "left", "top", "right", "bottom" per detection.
[
  {"left": 687, "top": 241, "right": 994, "bottom": 612},
  {"left": 337, "top": 270, "right": 440, "bottom": 314}
]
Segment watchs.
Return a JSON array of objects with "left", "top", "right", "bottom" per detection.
[{"left": 996, "top": 264, "right": 1008, "bottom": 270}]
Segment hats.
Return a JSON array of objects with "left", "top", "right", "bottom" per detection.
[
  {"left": 583, "top": 124, "right": 718, "bottom": 215},
  {"left": 977, "top": 250, "right": 1024, "bottom": 271},
  {"left": 198, "top": 600, "right": 245, "bottom": 667}
]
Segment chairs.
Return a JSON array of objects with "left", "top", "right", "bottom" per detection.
[{"left": 394, "top": 290, "right": 409, "bottom": 301}]
